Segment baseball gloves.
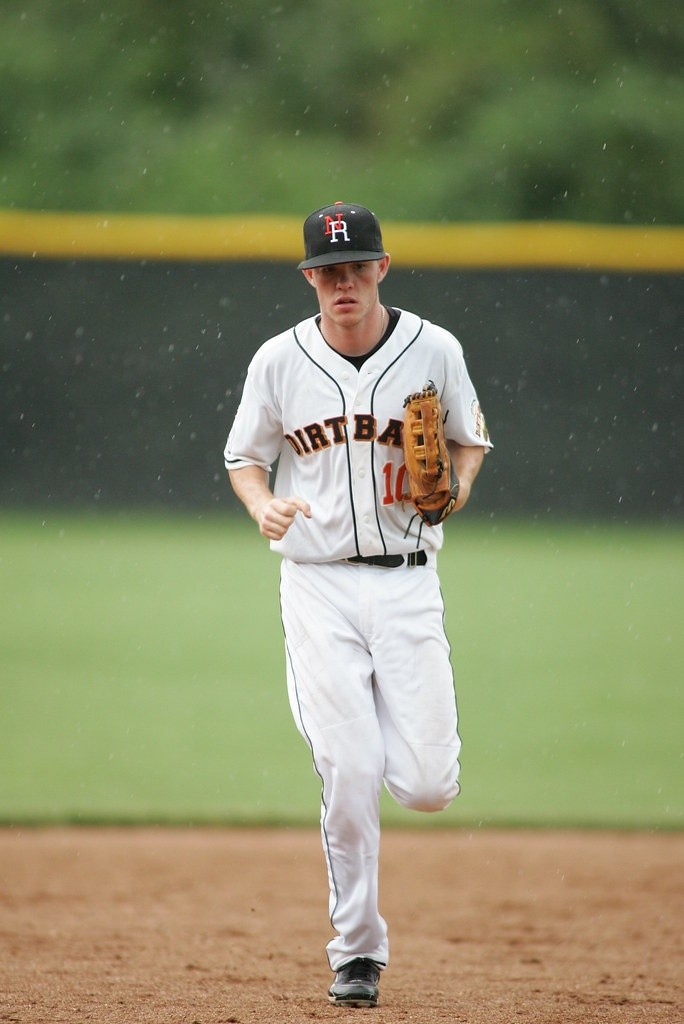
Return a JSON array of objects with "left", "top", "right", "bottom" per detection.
[{"left": 401, "top": 379, "right": 460, "bottom": 550}]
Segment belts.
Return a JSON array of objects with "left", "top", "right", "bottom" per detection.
[{"left": 340, "top": 549, "right": 427, "bottom": 568}]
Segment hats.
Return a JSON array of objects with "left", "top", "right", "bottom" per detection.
[{"left": 297, "top": 201, "right": 386, "bottom": 271}]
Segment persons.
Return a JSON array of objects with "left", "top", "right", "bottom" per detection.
[{"left": 219, "top": 207, "right": 493, "bottom": 1007}]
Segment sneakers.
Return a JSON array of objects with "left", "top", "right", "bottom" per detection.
[{"left": 328, "top": 957, "right": 386, "bottom": 1008}]
